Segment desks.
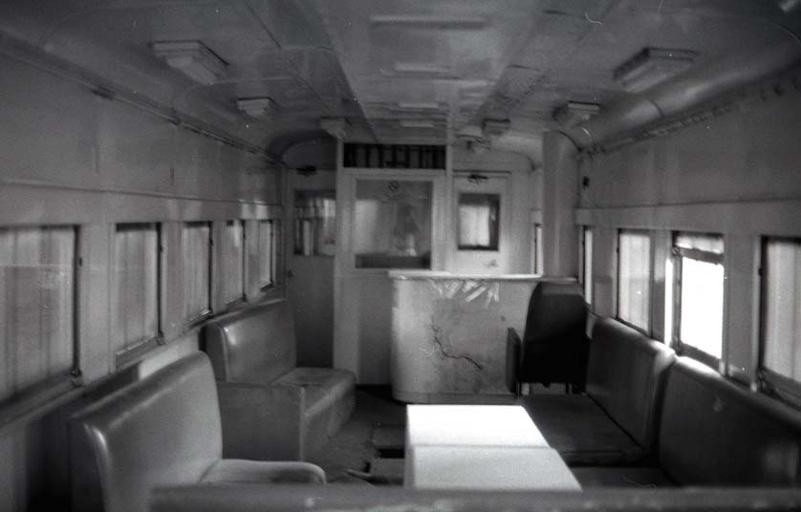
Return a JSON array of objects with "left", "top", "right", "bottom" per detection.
[{"left": 403, "top": 404, "right": 584, "bottom": 492}]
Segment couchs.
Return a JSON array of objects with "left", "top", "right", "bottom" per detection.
[
  {"left": 202, "top": 295, "right": 356, "bottom": 471},
  {"left": 69, "top": 351, "right": 326, "bottom": 510},
  {"left": 515, "top": 314, "right": 679, "bottom": 468},
  {"left": 143, "top": 485, "right": 797, "bottom": 509},
  {"left": 568, "top": 355, "right": 800, "bottom": 490}
]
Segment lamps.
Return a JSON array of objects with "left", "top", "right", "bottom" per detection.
[
  {"left": 320, "top": 118, "right": 354, "bottom": 142},
  {"left": 553, "top": 102, "right": 602, "bottom": 130},
  {"left": 609, "top": 45, "right": 699, "bottom": 96},
  {"left": 236, "top": 98, "right": 288, "bottom": 129},
  {"left": 155, "top": 42, "right": 232, "bottom": 91}
]
